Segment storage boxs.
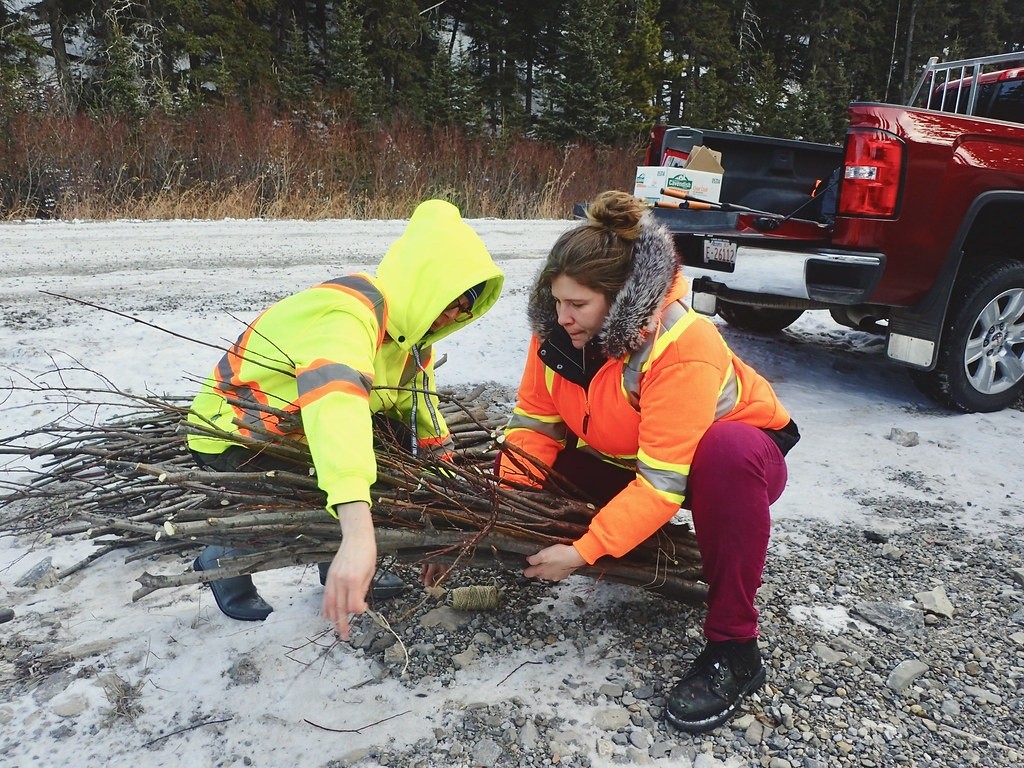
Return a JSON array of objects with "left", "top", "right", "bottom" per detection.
[{"left": 634, "top": 165, "right": 724, "bottom": 207}]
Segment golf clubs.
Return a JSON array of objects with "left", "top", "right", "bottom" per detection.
[
  {"left": 651, "top": 184, "right": 824, "bottom": 226},
  {"left": 749, "top": 178, "right": 843, "bottom": 234}
]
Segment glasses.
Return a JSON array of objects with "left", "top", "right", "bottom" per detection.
[{"left": 446, "top": 299, "right": 474, "bottom": 323}]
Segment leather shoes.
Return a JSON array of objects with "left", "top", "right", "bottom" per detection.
[
  {"left": 192, "top": 539, "right": 274, "bottom": 621},
  {"left": 319, "top": 558, "right": 408, "bottom": 596}
]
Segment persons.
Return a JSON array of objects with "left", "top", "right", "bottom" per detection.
[
  {"left": 186, "top": 199, "right": 505, "bottom": 641},
  {"left": 494, "top": 190, "right": 800, "bottom": 735}
]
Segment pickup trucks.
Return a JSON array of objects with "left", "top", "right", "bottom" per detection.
[{"left": 576, "top": 52, "right": 1023, "bottom": 414}]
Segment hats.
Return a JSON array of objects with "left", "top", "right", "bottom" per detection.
[{"left": 465, "top": 281, "right": 488, "bottom": 305}]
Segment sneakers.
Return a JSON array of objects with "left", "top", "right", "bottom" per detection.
[{"left": 666, "top": 639, "right": 766, "bottom": 731}]
